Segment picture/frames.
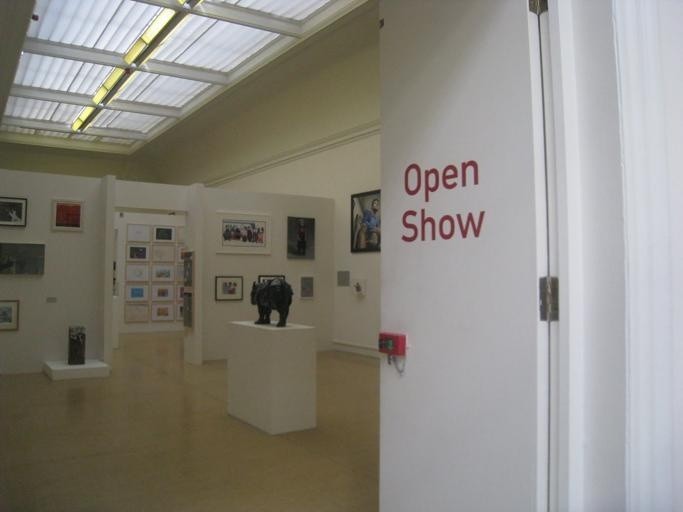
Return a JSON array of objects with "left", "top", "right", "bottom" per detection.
[
  {"left": 0, "top": 196, "right": 85, "bottom": 333},
  {"left": 349, "top": 189, "right": 382, "bottom": 254},
  {"left": 214, "top": 215, "right": 284, "bottom": 301},
  {"left": 123, "top": 222, "right": 193, "bottom": 325}
]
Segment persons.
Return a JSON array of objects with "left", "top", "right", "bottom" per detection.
[
  {"left": 227, "top": 282, "right": 232, "bottom": 289},
  {"left": 223, "top": 282, "right": 228, "bottom": 293},
  {"left": 295, "top": 219, "right": 306, "bottom": 256},
  {"left": 223, "top": 225, "right": 263, "bottom": 243},
  {"left": 229, "top": 282, "right": 237, "bottom": 294},
  {"left": 361, "top": 198, "right": 381, "bottom": 251}
]
